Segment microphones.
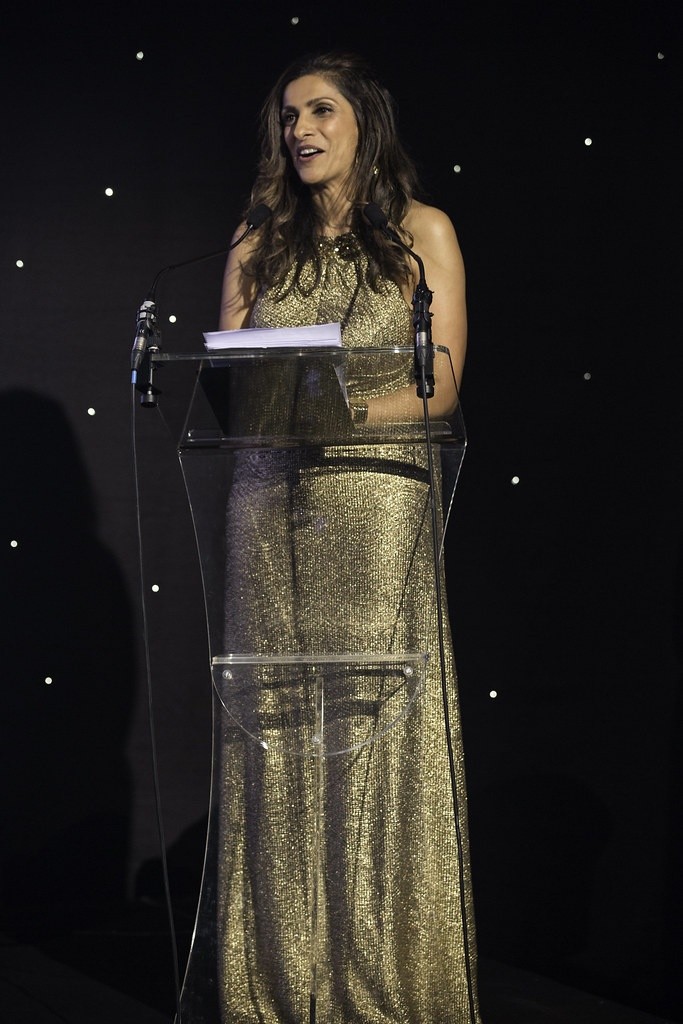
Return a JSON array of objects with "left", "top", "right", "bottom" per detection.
[
  {"left": 364, "top": 201, "right": 431, "bottom": 368},
  {"left": 130, "top": 202, "right": 272, "bottom": 372}
]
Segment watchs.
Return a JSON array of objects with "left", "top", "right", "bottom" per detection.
[{"left": 348, "top": 399, "right": 369, "bottom": 423}]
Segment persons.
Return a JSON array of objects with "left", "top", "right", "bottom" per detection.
[{"left": 216, "top": 51, "right": 481, "bottom": 1024}]
